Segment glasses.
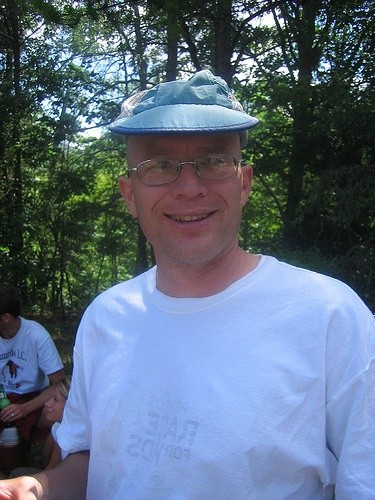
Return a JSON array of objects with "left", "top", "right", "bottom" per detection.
[{"left": 128, "top": 154, "right": 244, "bottom": 186}]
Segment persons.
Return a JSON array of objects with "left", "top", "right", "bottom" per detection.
[
  {"left": 0, "top": 70, "right": 375, "bottom": 500},
  {"left": 0, "top": 290, "right": 76, "bottom": 481}
]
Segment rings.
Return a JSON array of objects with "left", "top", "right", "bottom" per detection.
[{"left": 10, "top": 412, "right": 15, "bottom": 416}]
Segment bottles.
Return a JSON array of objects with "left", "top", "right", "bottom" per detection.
[{"left": 0, "top": 384, "right": 16, "bottom": 428}]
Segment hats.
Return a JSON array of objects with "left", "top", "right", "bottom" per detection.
[{"left": 108, "top": 70, "right": 260, "bottom": 149}]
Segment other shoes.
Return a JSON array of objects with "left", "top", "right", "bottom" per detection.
[{"left": 27, "top": 462, "right": 47, "bottom": 469}]
